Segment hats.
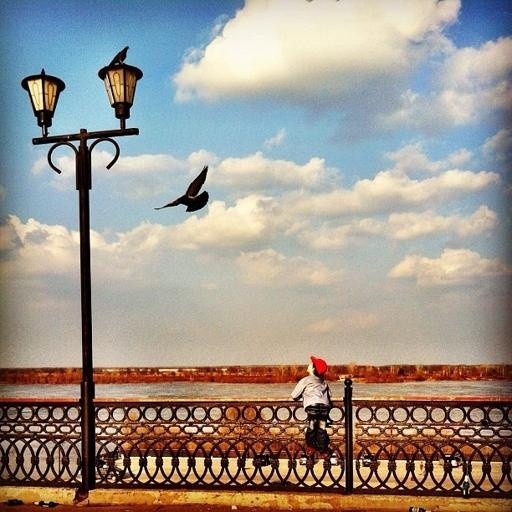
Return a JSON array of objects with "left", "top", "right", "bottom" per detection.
[{"left": 311, "top": 356, "right": 327, "bottom": 375}]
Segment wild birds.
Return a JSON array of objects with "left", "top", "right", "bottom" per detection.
[{"left": 155, "top": 164, "right": 209, "bottom": 212}]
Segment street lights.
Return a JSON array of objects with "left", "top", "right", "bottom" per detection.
[{"left": 22, "top": 60, "right": 143, "bottom": 492}]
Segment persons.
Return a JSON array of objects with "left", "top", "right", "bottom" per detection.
[{"left": 292, "top": 357, "right": 331, "bottom": 448}]
